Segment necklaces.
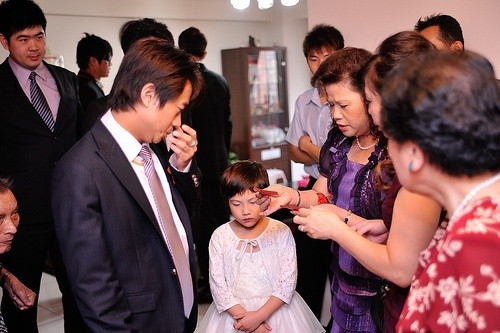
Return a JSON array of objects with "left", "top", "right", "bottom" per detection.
[
  {"left": 132, "top": 160, "right": 144, "bottom": 166},
  {"left": 356, "top": 136, "right": 379, "bottom": 149},
  {"left": 448, "top": 173, "right": 500, "bottom": 225}
]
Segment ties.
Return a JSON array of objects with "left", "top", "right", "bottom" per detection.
[
  {"left": 140, "top": 145, "right": 194, "bottom": 318},
  {"left": 28, "top": 72, "right": 55, "bottom": 132}
]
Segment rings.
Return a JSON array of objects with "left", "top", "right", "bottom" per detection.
[
  {"left": 190, "top": 141, "right": 196, "bottom": 147},
  {"left": 299, "top": 226, "right": 304, "bottom": 231}
]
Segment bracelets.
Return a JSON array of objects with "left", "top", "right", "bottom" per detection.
[
  {"left": 296, "top": 190, "right": 301, "bottom": 207},
  {"left": 344, "top": 210, "right": 351, "bottom": 224}
]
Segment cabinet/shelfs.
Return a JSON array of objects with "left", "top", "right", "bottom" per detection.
[{"left": 222, "top": 46, "right": 291, "bottom": 185}]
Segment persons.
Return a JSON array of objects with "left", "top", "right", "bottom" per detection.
[
  {"left": 0, "top": 176, "right": 37, "bottom": 333},
  {"left": 379, "top": 49, "right": 500, "bottom": 333},
  {"left": 178, "top": 27, "right": 233, "bottom": 305},
  {"left": 0, "top": 0, "right": 87, "bottom": 333},
  {"left": 76, "top": 32, "right": 113, "bottom": 114},
  {"left": 292, "top": 30, "right": 451, "bottom": 333},
  {"left": 412, "top": 12, "right": 466, "bottom": 56},
  {"left": 255, "top": 46, "right": 396, "bottom": 333},
  {"left": 201, "top": 159, "right": 326, "bottom": 333},
  {"left": 284, "top": 24, "right": 345, "bottom": 329},
  {"left": 50, "top": 37, "right": 203, "bottom": 333},
  {"left": 119, "top": 18, "right": 174, "bottom": 57}
]
